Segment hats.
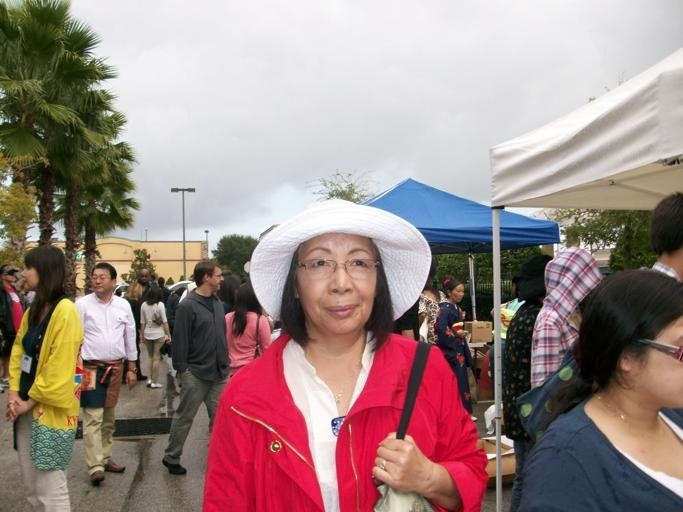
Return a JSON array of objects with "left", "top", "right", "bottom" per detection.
[
  {"left": 247, "top": 196, "right": 433, "bottom": 327},
  {"left": 0, "top": 264, "right": 20, "bottom": 277}
]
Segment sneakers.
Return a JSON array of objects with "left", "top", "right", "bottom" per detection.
[
  {"left": 470, "top": 415, "right": 477, "bottom": 421},
  {"left": 121, "top": 374, "right": 163, "bottom": 389}
]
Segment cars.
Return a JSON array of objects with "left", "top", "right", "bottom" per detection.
[
  {"left": 167, "top": 280, "right": 196, "bottom": 292},
  {"left": 113, "top": 283, "right": 129, "bottom": 298}
]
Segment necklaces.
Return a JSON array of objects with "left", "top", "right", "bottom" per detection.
[
  {"left": 306, "top": 348, "right": 360, "bottom": 405},
  {"left": 592, "top": 393, "right": 663, "bottom": 440}
]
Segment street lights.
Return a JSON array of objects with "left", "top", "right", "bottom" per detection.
[{"left": 169, "top": 186, "right": 195, "bottom": 278}]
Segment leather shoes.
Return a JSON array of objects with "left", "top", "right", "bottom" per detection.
[
  {"left": 104, "top": 458, "right": 126, "bottom": 473},
  {"left": 161, "top": 458, "right": 187, "bottom": 475},
  {"left": 91, "top": 469, "right": 105, "bottom": 487}
]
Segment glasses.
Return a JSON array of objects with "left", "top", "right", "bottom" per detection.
[{"left": 293, "top": 258, "right": 380, "bottom": 278}]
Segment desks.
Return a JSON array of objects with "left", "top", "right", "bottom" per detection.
[{"left": 478, "top": 437, "right": 515, "bottom": 488}]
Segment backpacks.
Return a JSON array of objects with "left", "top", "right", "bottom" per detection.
[{"left": 511, "top": 340, "right": 595, "bottom": 446}]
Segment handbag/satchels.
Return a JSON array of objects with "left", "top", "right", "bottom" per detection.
[
  {"left": 152, "top": 312, "right": 163, "bottom": 327},
  {"left": 373, "top": 340, "right": 435, "bottom": 512}
]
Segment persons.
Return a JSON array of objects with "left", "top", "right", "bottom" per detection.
[
  {"left": 502, "top": 255, "right": 553, "bottom": 512},
  {"left": 530, "top": 247, "right": 604, "bottom": 391},
  {"left": 518, "top": 271, "right": 683, "bottom": 512},
  {"left": 417, "top": 276, "right": 527, "bottom": 422},
  {"left": 75, "top": 263, "right": 138, "bottom": 486},
  {"left": 5, "top": 244, "right": 83, "bottom": 512},
  {"left": 639, "top": 193, "right": 683, "bottom": 285},
  {"left": 0, "top": 263, "right": 35, "bottom": 393},
  {"left": 113, "top": 268, "right": 275, "bottom": 388},
  {"left": 201, "top": 198, "right": 489, "bottom": 512},
  {"left": 162, "top": 262, "right": 231, "bottom": 475}
]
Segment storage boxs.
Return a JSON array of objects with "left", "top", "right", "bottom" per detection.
[{"left": 464, "top": 320, "right": 493, "bottom": 343}]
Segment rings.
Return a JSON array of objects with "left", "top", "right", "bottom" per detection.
[{"left": 379, "top": 460, "right": 386, "bottom": 469}]
